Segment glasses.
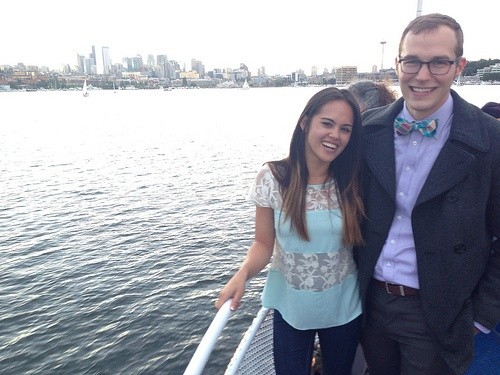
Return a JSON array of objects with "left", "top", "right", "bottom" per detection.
[{"left": 397, "top": 56, "right": 460, "bottom": 75}]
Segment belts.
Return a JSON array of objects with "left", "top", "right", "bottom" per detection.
[{"left": 368, "top": 276, "right": 418, "bottom": 297}]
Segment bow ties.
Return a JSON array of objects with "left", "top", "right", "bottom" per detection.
[{"left": 395, "top": 117, "right": 439, "bottom": 137}]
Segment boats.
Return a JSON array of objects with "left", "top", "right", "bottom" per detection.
[
  {"left": 465, "top": 79, "right": 500, "bottom": 85},
  {"left": 126, "top": 85, "right": 139, "bottom": 90}
]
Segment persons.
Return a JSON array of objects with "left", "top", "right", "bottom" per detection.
[
  {"left": 354, "top": 14, "right": 500, "bottom": 375},
  {"left": 349, "top": 81, "right": 396, "bottom": 112},
  {"left": 216, "top": 87, "right": 368, "bottom": 375}
]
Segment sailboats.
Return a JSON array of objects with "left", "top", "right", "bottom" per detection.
[{"left": 83, "top": 79, "right": 89, "bottom": 97}]
